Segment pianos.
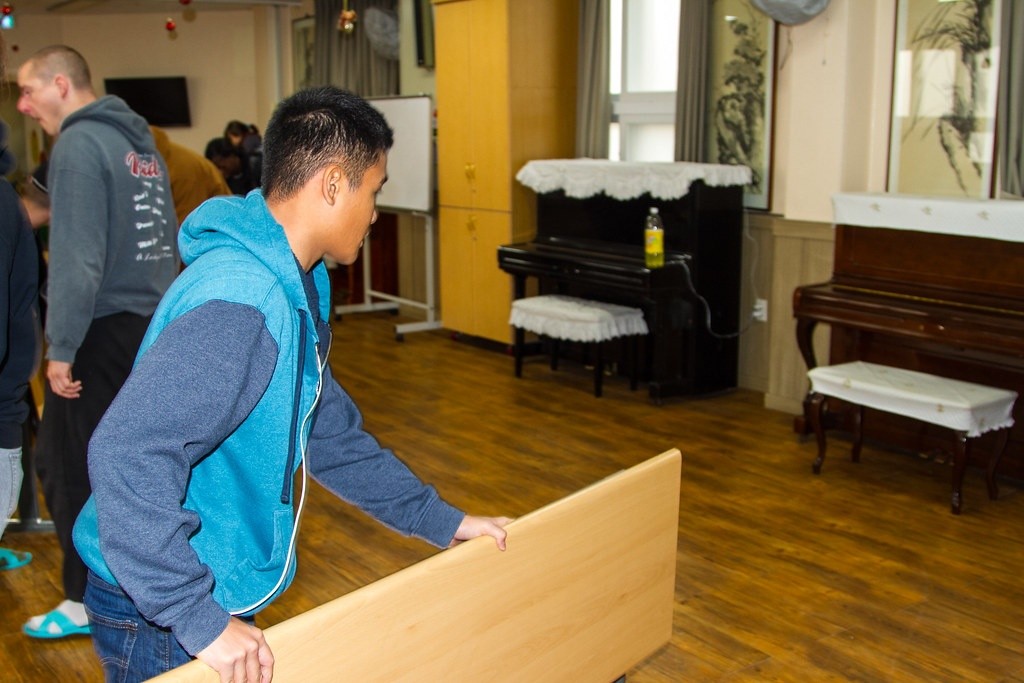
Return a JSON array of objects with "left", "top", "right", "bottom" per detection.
[
  {"left": 790, "top": 191, "right": 1024, "bottom": 484},
  {"left": 497, "top": 155, "right": 750, "bottom": 400}
]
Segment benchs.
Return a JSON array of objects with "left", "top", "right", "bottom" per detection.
[
  {"left": 807, "top": 359, "right": 1019, "bottom": 516},
  {"left": 508, "top": 294, "right": 649, "bottom": 400}
]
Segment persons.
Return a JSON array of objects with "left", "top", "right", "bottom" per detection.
[
  {"left": 0, "top": 120, "right": 57, "bottom": 569},
  {"left": 149, "top": 118, "right": 263, "bottom": 224},
  {"left": 16, "top": 47, "right": 182, "bottom": 638},
  {"left": 73, "top": 84, "right": 513, "bottom": 683}
]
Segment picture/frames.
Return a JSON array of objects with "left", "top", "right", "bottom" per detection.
[
  {"left": 707, "top": 0, "right": 778, "bottom": 211},
  {"left": 290, "top": 14, "right": 314, "bottom": 96},
  {"left": 885, "top": 1, "right": 1003, "bottom": 200}
]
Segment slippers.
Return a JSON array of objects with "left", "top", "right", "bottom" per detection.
[
  {"left": 24, "top": 607, "right": 91, "bottom": 638},
  {"left": 0, "top": 547, "right": 33, "bottom": 571}
]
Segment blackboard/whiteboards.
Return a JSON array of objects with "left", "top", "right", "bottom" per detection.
[{"left": 362, "top": 94, "right": 434, "bottom": 217}]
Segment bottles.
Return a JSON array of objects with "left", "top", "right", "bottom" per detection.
[{"left": 644, "top": 207, "right": 665, "bottom": 269}]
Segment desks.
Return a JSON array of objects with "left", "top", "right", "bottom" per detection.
[{"left": 144, "top": 448, "right": 683, "bottom": 683}]
